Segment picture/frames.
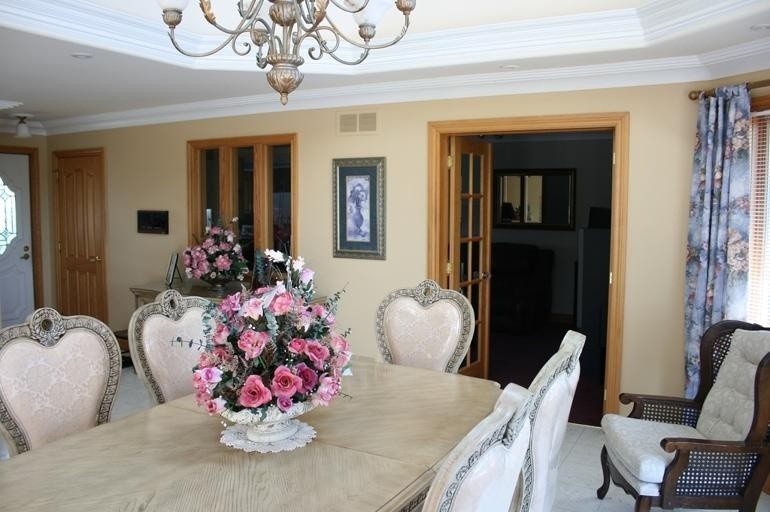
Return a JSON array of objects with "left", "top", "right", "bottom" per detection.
[
  {"left": 163, "top": 249, "right": 181, "bottom": 285},
  {"left": 327, "top": 154, "right": 390, "bottom": 263}
]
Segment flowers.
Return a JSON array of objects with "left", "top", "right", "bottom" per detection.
[
  {"left": 182, "top": 217, "right": 248, "bottom": 280},
  {"left": 257, "top": 248, "right": 315, "bottom": 294}
]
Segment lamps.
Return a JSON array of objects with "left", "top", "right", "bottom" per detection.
[
  {"left": 154, "top": 1, "right": 418, "bottom": 110},
  {"left": 11, "top": 112, "right": 36, "bottom": 143}
]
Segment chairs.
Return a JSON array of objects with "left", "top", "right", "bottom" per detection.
[
  {"left": 460, "top": 241, "right": 560, "bottom": 333},
  {"left": 593, "top": 316, "right": 770, "bottom": 512}
]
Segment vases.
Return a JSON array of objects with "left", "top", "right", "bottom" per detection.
[{"left": 200, "top": 275, "right": 232, "bottom": 290}]
